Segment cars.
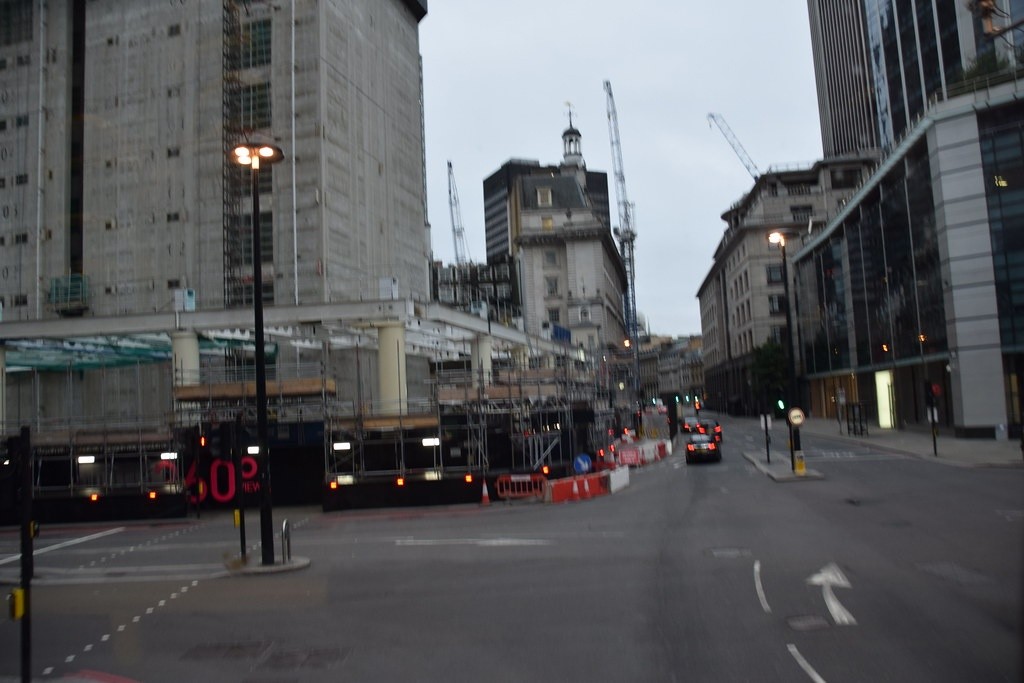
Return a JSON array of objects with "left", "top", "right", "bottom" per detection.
[{"left": 680, "top": 415, "right": 722, "bottom": 465}]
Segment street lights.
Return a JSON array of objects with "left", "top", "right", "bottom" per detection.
[
  {"left": 228, "top": 142, "right": 286, "bottom": 566},
  {"left": 769, "top": 232, "right": 800, "bottom": 451}
]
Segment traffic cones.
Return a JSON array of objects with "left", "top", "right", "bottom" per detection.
[
  {"left": 570, "top": 476, "right": 593, "bottom": 503},
  {"left": 640, "top": 449, "right": 647, "bottom": 465},
  {"left": 480, "top": 479, "right": 491, "bottom": 505}
]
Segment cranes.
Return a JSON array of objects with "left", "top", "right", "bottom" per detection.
[
  {"left": 707, "top": 111, "right": 761, "bottom": 181},
  {"left": 448, "top": 161, "right": 473, "bottom": 310},
  {"left": 603, "top": 79, "right": 641, "bottom": 396}
]
